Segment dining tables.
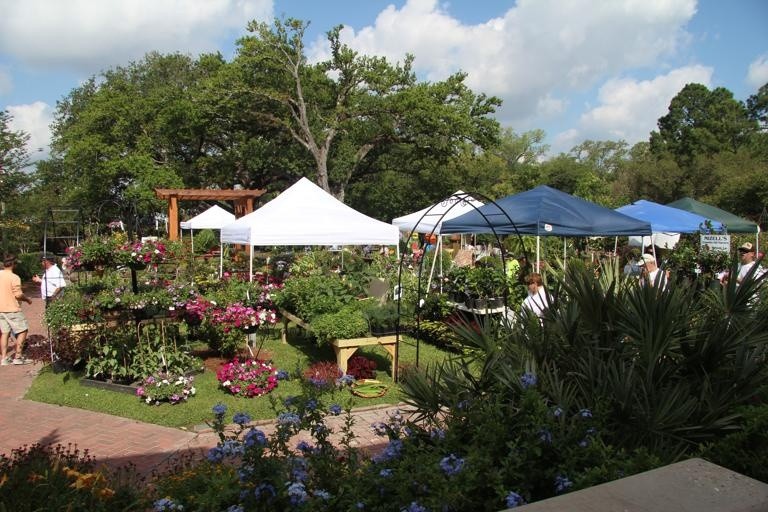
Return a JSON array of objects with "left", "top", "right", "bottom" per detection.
[{"left": 450, "top": 266, "right": 507, "bottom": 310}]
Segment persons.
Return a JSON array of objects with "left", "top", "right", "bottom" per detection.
[
  {"left": 31, "top": 255, "right": 67, "bottom": 312},
  {"left": 720, "top": 240, "right": 766, "bottom": 313},
  {"left": 634, "top": 252, "right": 668, "bottom": 296},
  {"left": 0, "top": 251, "right": 32, "bottom": 366},
  {"left": 518, "top": 270, "right": 555, "bottom": 325}
]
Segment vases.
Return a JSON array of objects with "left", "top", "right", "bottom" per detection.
[{"left": 66, "top": 234, "right": 279, "bottom": 404}]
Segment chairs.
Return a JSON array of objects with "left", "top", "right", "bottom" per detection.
[
  {"left": 1, "top": 356, "right": 14, "bottom": 366},
  {"left": 13, "top": 357, "right": 34, "bottom": 365}
]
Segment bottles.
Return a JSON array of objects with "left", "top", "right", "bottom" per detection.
[
  {"left": 636, "top": 254, "right": 654, "bottom": 266},
  {"left": 738, "top": 242, "right": 754, "bottom": 252}
]
